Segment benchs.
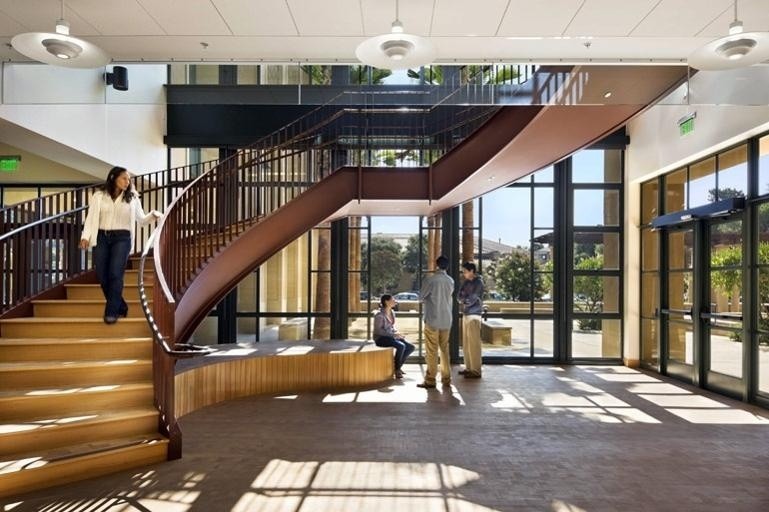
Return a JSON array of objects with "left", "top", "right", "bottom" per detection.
[{"left": 280, "top": 318, "right": 308, "bottom": 342}]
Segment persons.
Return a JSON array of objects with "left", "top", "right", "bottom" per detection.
[
  {"left": 456, "top": 259, "right": 484, "bottom": 379},
  {"left": 373, "top": 293, "right": 415, "bottom": 379},
  {"left": 76, "top": 166, "right": 165, "bottom": 324},
  {"left": 415, "top": 253, "right": 456, "bottom": 388}
]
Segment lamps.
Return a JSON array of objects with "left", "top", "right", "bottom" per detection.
[
  {"left": 688, "top": 0, "right": 769, "bottom": 72},
  {"left": 356, "top": 0, "right": 435, "bottom": 71},
  {"left": 12, "top": 0, "right": 112, "bottom": 69}
]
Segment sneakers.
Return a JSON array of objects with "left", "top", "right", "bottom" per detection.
[
  {"left": 415, "top": 374, "right": 452, "bottom": 388},
  {"left": 457, "top": 367, "right": 484, "bottom": 380},
  {"left": 394, "top": 367, "right": 406, "bottom": 379},
  {"left": 102, "top": 302, "right": 130, "bottom": 325}
]
